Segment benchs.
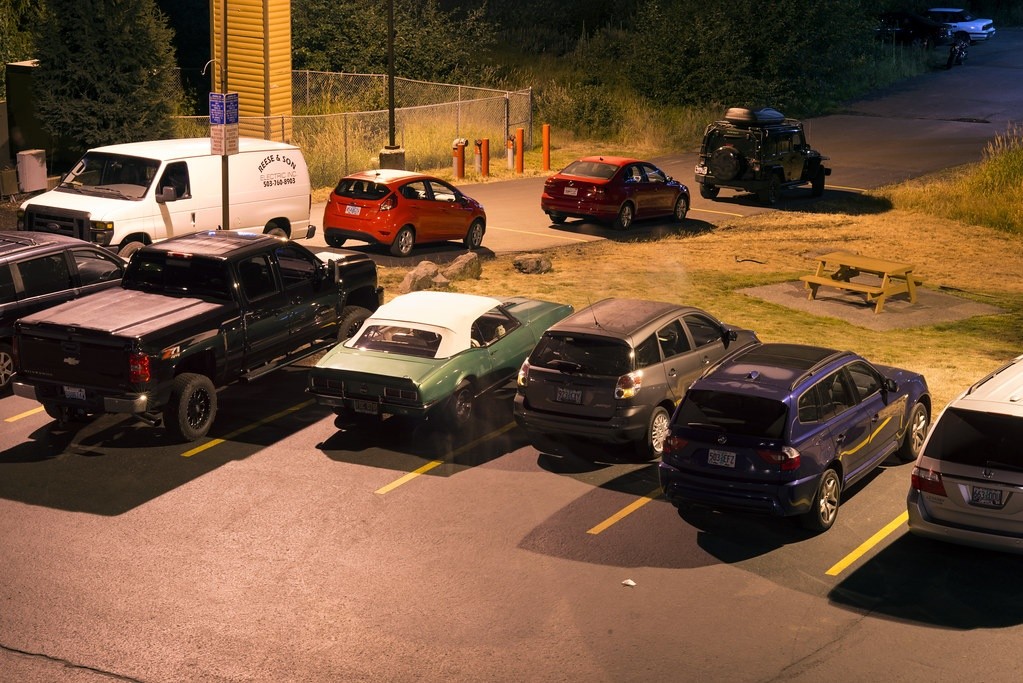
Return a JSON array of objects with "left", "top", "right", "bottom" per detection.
[
  {"left": 799, "top": 274, "right": 885, "bottom": 295},
  {"left": 853, "top": 268, "right": 929, "bottom": 286}
]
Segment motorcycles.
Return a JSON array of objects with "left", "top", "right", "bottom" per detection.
[{"left": 947, "top": 39, "right": 969, "bottom": 69}]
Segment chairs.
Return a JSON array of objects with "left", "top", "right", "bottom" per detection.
[
  {"left": 804, "top": 384, "right": 842, "bottom": 419},
  {"left": 641, "top": 336, "right": 677, "bottom": 365},
  {"left": 407, "top": 187, "right": 418, "bottom": 199},
  {"left": 597, "top": 165, "right": 634, "bottom": 180},
  {"left": 244, "top": 263, "right": 269, "bottom": 295}
]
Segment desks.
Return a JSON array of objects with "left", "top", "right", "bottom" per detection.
[{"left": 808, "top": 251, "right": 917, "bottom": 313}]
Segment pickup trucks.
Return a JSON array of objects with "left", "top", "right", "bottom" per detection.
[{"left": 12, "top": 230, "right": 384, "bottom": 442}]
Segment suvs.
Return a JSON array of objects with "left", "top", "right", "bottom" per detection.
[
  {"left": 659, "top": 344, "right": 932, "bottom": 530},
  {"left": 695, "top": 107, "right": 832, "bottom": 204},
  {"left": 514, "top": 296, "right": 760, "bottom": 459},
  {"left": 0, "top": 231, "right": 128, "bottom": 397}
]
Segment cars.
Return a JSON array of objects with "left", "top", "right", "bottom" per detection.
[
  {"left": 875, "top": 12, "right": 954, "bottom": 49},
  {"left": 928, "top": 8, "right": 996, "bottom": 44},
  {"left": 309, "top": 290, "right": 574, "bottom": 428},
  {"left": 323, "top": 169, "right": 487, "bottom": 258},
  {"left": 541, "top": 156, "right": 690, "bottom": 229}
]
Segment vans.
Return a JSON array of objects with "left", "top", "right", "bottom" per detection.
[
  {"left": 17, "top": 137, "right": 316, "bottom": 259},
  {"left": 907, "top": 354, "right": 1023, "bottom": 554}
]
